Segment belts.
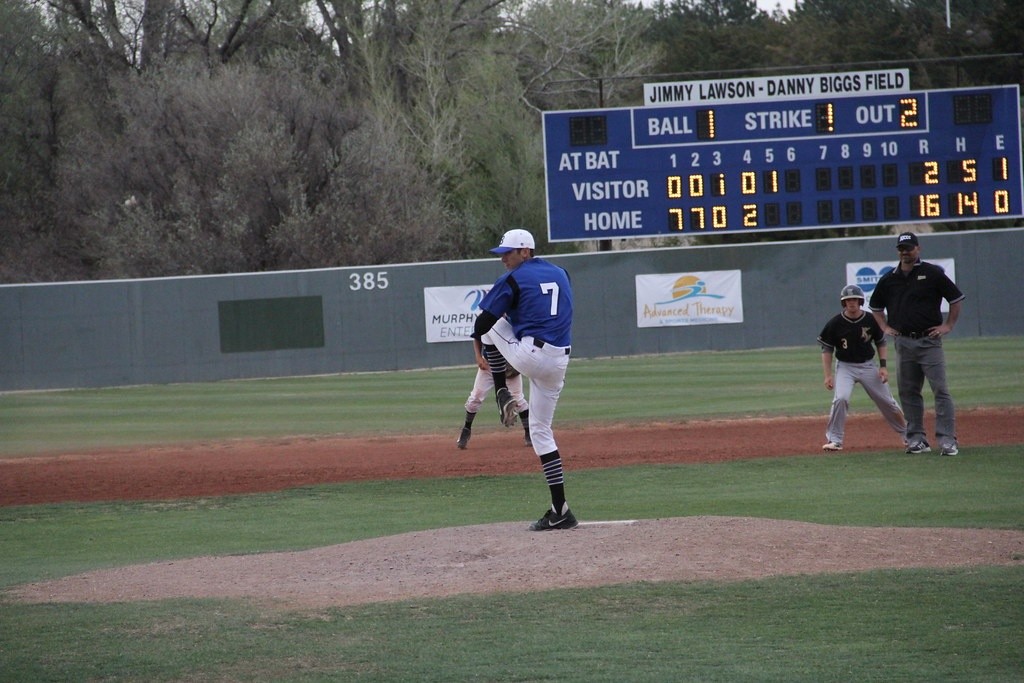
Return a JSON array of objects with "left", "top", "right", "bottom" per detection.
[
  {"left": 534, "top": 338, "right": 570, "bottom": 354},
  {"left": 897, "top": 331, "right": 929, "bottom": 340}
]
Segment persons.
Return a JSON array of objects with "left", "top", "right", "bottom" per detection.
[
  {"left": 868, "top": 232, "right": 966, "bottom": 455},
  {"left": 817, "top": 285, "right": 910, "bottom": 451},
  {"left": 457, "top": 229, "right": 579, "bottom": 531}
]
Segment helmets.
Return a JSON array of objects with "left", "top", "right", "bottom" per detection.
[{"left": 840, "top": 284, "right": 864, "bottom": 309}]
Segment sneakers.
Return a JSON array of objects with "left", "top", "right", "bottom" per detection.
[
  {"left": 940, "top": 441, "right": 958, "bottom": 455},
  {"left": 497, "top": 388, "right": 518, "bottom": 428},
  {"left": 528, "top": 501, "right": 580, "bottom": 531},
  {"left": 905, "top": 437, "right": 931, "bottom": 454},
  {"left": 457, "top": 427, "right": 471, "bottom": 448},
  {"left": 822, "top": 441, "right": 843, "bottom": 451},
  {"left": 902, "top": 440, "right": 909, "bottom": 448},
  {"left": 524, "top": 433, "right": 532, "bottom": 446}
]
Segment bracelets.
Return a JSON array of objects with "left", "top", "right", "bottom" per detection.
[{"left": 880, "top": 359, "right": 886, "bottom": 367}]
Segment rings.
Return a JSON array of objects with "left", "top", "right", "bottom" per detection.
[{"left": 936, "top": 332, "right": 939, "bottom": 334}]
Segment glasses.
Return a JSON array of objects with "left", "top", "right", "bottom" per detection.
[{"left": 898, "top": 246, "right": 914, "bottom": 251}]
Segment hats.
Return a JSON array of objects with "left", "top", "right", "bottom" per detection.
[
  {"left": 489, "top": 229, "right": 535, "bottom": 254},
  {"left": 896, "top": 232, "right": 918, "bottom": 247}
]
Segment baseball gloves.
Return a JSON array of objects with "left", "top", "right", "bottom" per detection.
[{"left": 506, "top": 363, "right": 520, "bottom": 379}]
